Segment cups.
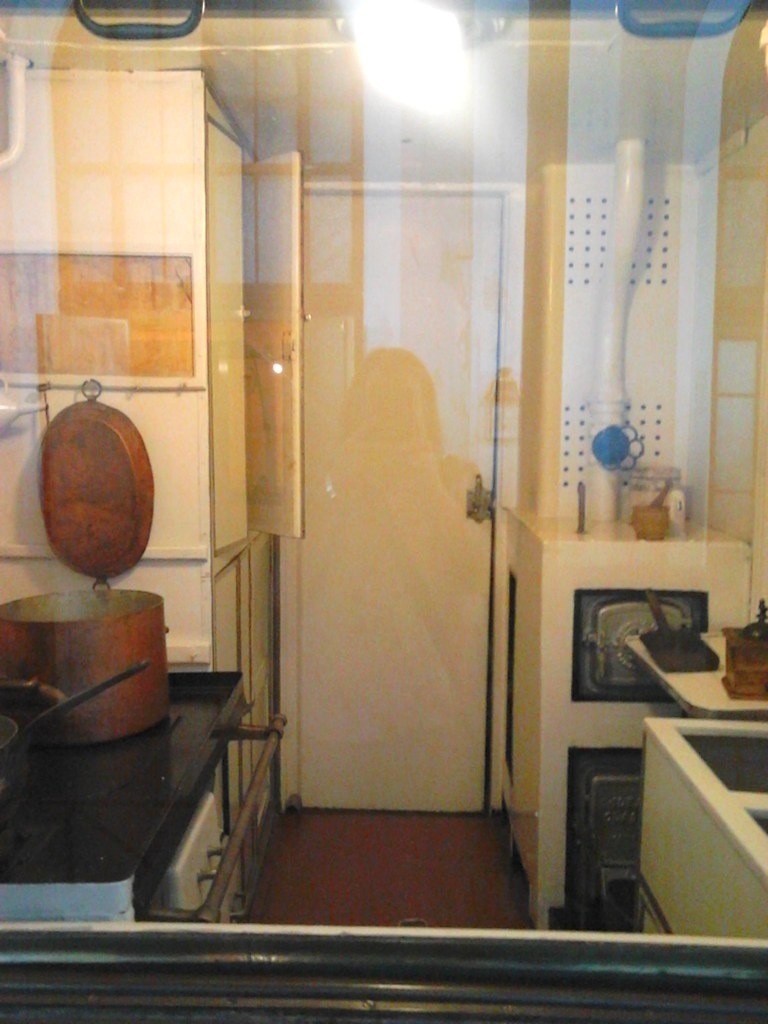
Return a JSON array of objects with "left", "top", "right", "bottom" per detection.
[{"left": 631, "top": 505, "right": 669, "bottom": 541}]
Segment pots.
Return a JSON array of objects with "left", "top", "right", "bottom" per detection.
[
  {"left": 39, "top": 379, "right": 155, "bottom": 590},
  {"left": 0, "top": 587, "right": 169, "bottom": 749},
  {"left": 0, "top": 659, "right": 152, "bottom": 814}
]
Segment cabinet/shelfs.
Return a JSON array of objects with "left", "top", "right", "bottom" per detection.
[
  {"left": 503, "top": 506, "right": 757, "bottom": 923},
  {"left": 0, "top": 69, "right": 306, "bottom": 923}
]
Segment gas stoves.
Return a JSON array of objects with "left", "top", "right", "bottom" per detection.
[{"left": 0, "top": 670, "right": 252, "bottom": 920}]
